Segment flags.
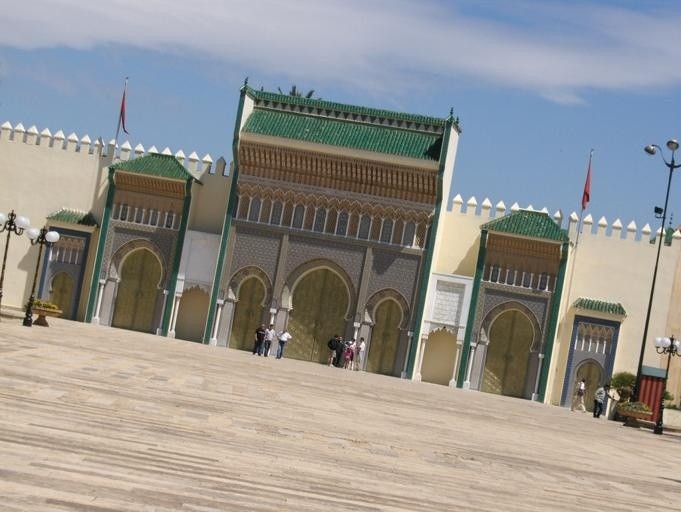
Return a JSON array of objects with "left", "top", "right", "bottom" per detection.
[
  {"left": 582, "top": 148, "right": 594, "bottom": 209},
  {"left": 121, "top": 77, "right": 129, "bottom": 134}
]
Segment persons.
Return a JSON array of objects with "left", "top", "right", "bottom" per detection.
[
  {"left": 253, "top": 324, "right": 293, "bottom": 359},
  {"left": 594, "top": 384, "right": 615, "bottom": 418},
  {"left": 327, "top": 334, "right": 366, "bottom": 371},
  {"left": 571, "top": 378, "right": 586, "bottom": 412}
]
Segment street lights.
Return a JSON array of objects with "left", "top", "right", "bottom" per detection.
[
  {"left": 631, "top": 138, "right": 680, "bottom": 408},
  {"left": 0, "top": 210, "right": 29, "bottom": 300},
  {"left": 652, "top": 334, "right": 680, "bottom": 435},
  {"left": 19, "top": 226, "right": 59, "bottom": 327}
]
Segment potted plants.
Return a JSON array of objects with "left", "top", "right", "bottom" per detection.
[
  {"left": 609, "top": 372, "right": 637, "bottom": 420},
  {"left": 616, "top": 402, "right": 652, "bottom": 429},
  {"left": 25, "top": 301, "right": 63, "bottom": 327}
]
